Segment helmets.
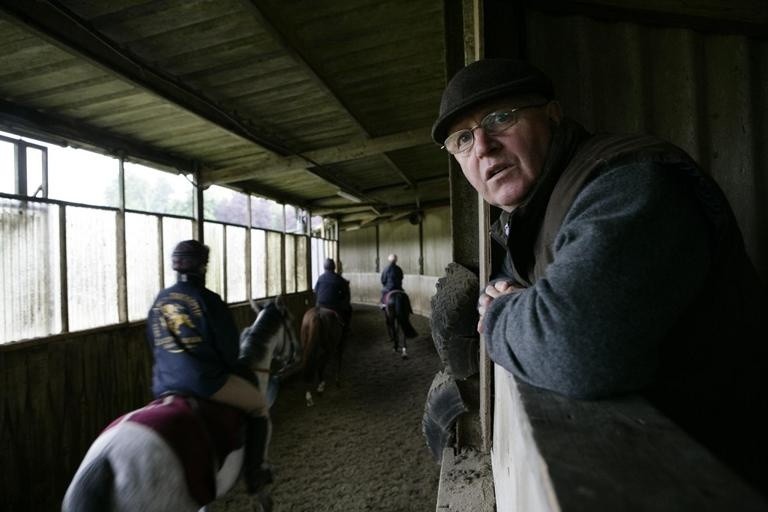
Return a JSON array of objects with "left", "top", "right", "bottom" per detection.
[
  {"left": 387, "top": 254, "right": 398, "bottom": 263},
  {"left": 173, "top": 240, "right": 209, "bottom": 274},
  {"left": 323, "top": 258, "right": 335, "bottom": 270}
]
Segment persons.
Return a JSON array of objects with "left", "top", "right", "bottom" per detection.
[
  {"left": 313, "top": 257, "right": 352, "bottom": 335},
  {"left": 380, "top": 254, "right": 405, "bottom": 311},
  {"left": 147, "top": 240, "right": 275, "bottom": 495},
  {"left": 432, "top": 59, "right": 767, "bottom": 499}
]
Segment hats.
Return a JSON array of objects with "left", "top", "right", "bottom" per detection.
[{"left": 431, "top": 58, "right": 556, "bottom": 147}]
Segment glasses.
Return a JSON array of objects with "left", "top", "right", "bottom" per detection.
[{"left": 440, "top": 102, "right": 547, "bottom": 155}]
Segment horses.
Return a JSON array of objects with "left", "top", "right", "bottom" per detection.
[
  {"left": 300, "top": 305, "right": 343, "bottom": 407},
  {"left": 61, "top": 295, "right": 299, "bottom": 512},
  {"left": 382, "top": 289, "right": 410, "bottom": 357}
]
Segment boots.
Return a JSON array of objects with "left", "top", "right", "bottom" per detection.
[{"left": 243, "top": 415, "right": 274, "bottom": 497}]
